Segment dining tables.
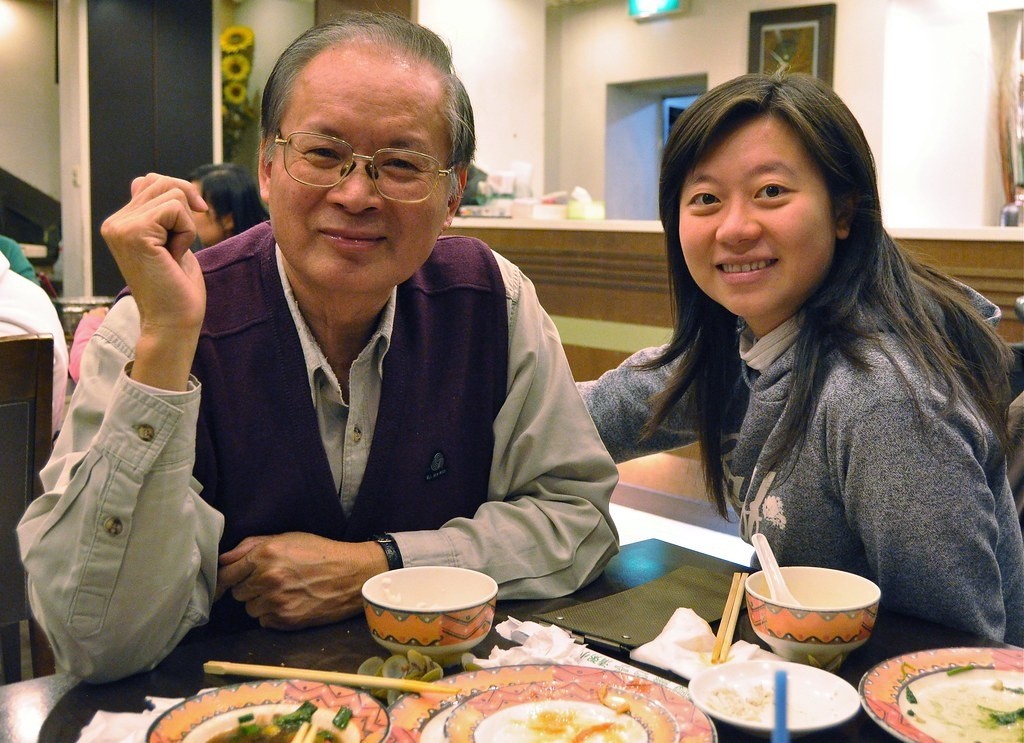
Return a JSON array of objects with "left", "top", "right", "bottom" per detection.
[{"left": 0, "top": 538, "right": 903, "bottom": 743}]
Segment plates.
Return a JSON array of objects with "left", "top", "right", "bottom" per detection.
[
  {"left": 687, "top": 658, "right": 864, "bottom": 743},
  {"left": 385, "top": 663, "right": 719, "bottom": 743},
  {"left": 856, "top": 646, "right": 1024, "bottom": 743},
  {"left": 144, "top": 678, "right": 392, "bottom": 743}
]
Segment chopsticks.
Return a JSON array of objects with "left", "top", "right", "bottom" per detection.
[
  {"left": 710, "top": 572, "right": 750, "bottom": 663},
  {"left": 201, "top": 660, "right": 462, "bottom": 696}
]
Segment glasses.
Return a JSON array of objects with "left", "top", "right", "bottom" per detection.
[{"left": 275, "top": 127, "right": 458, "bottom": 204}]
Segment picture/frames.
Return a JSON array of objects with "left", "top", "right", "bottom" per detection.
[{"left": 749, "top": 4, "right": 836, "bottom": 87}]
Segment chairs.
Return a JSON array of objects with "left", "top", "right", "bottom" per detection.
[{"left": 0, "top": 332, "right": 54, "bottom": 681}]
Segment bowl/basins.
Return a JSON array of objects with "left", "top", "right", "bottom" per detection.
[
  {"left": 359, "top": 564, "right": 499, "bottom": 670},
  {"left": 52, "top": 295, "right": 115, "bottom": 337},
  {"left": 744, "top": 565, "right": 881, "bottom": 675}
]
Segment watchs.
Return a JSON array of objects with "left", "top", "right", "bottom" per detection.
[{"left": 369, "top": 533, "right": 403, "bottom": 571}]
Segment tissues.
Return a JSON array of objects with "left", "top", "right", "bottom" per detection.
[{"left": 566, "top": 186, "right": 606, "bottom": 220}]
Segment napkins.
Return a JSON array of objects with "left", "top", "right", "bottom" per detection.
[{"left": 631, "top": 608, "right": 762, "bottom": 685}]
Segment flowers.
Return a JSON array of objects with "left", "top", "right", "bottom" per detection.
[{"left": 220, "top": 26, "right": 255, "bottom": 162}]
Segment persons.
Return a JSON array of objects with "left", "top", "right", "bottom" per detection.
[
  {"left": 0, "top": 163, "right": 270, "bottom": 450},
  {"left": 575, "top": 73, "right": 1024, "bottom": 648},
  {"left": 16, "top": 13, "right": 620, "bottom": 684}
]
